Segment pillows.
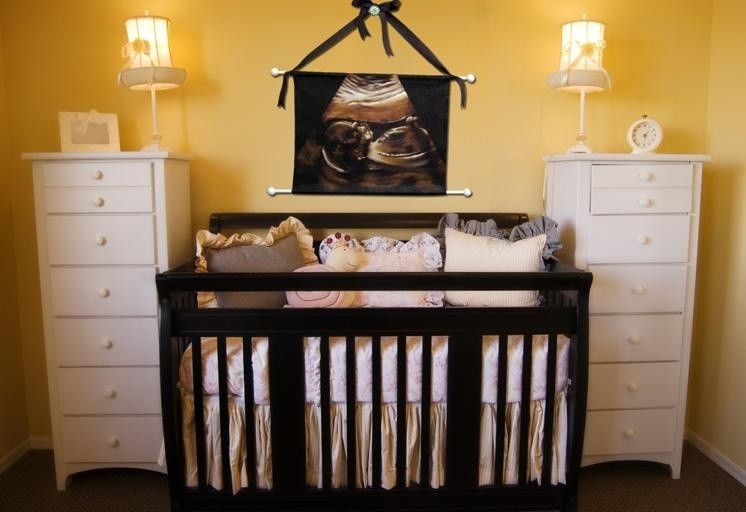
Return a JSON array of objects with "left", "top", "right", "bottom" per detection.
[
  {"left": 441, "top": 226, "right": 548, "bottom": 305},
  {"left": 430, "top": 214, "right": 563, "bottom": 270},
  {"left": 195, "top": 214, "right": 319, "bottom": 309},
  {"left": 321, "top": 233, "right": 444, "bottom": 308}
]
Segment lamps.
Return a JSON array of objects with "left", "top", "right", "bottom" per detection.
[
  {"left": 121, "top": 15, "right": 186, "bottom": 152},
  {"left": 551, "top": 20, "right": 611, "bottom": 154}
]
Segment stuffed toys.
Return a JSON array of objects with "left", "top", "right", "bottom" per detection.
[{"left": 283, "top": 244, "right": 366, "bottom": 309}]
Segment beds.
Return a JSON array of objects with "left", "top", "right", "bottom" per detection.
[{"left": 155, "top": 212, "right": 593, "bottom": 512}]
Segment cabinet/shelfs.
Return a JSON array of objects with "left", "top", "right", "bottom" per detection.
[
  {"left": 545, "top": 155, "right": 708, "bottom": 479},
  {"left": 20, "top": 152, "right": 196, "bottom": 492}
]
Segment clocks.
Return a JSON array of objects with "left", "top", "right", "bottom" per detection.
[{"left": 629, "top": 119, "right": 663, "bottom": 155}]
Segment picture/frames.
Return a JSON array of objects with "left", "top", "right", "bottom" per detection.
[{"left": 57, "top": 111, "right": 119, "bottom": 152}]
